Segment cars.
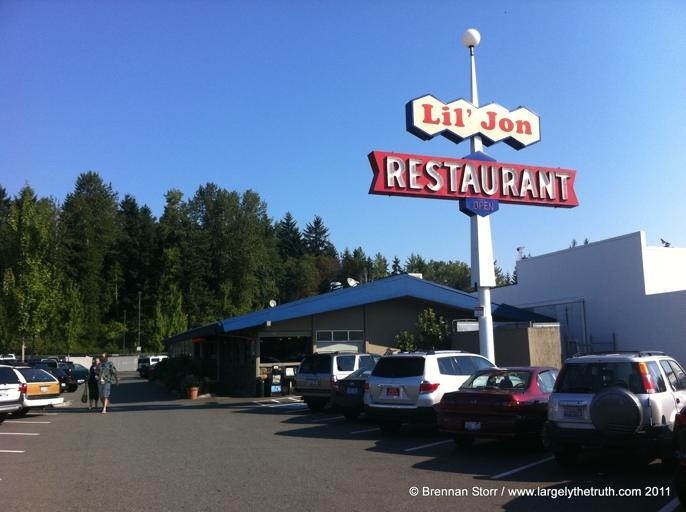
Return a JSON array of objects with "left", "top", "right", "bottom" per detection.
[
  {"left": 435, "top": 365, "right": 559, "bottom": 450},
  {"left": 330, "top": 367, "right": 376, "bottom": 422},
  {"left": 0, "top": 354, "right": 89, "bottom": 416}
]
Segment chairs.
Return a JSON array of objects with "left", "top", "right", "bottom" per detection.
[{"left": 499, "top": 379, "right": 514, "bottom": 388}]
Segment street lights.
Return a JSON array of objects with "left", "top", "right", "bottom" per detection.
[{"left": 464, "top": 28, "right": 495, "bottom": 366}]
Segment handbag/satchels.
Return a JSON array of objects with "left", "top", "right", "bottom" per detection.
[{"left": 81, "top": 393, "right": 89, "bottom": 403}]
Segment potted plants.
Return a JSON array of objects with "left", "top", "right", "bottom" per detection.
[{"left": 183, "top": 383, "right": 200, "bottom": 399}]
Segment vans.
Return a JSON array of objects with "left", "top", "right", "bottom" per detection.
[{"left": 138, "top": 356, "right": 168, "bottom": 378}]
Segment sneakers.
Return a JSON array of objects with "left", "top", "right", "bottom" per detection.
[
  {"left": 88, "top": 406, "right": 98, "bottom": 411},
  {"left": 101, "top": 408, "right": 107, "bottom": 413}
]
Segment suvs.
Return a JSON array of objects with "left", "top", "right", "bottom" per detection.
[
  {"left": 542, "top": 351, "right": 685, "bottom": 468},
  {"left": 292, "top": 351, "right": 381, "bottom": 411},
  {"left": 364, "top": 348, "right": 497, "bottom": 430}
]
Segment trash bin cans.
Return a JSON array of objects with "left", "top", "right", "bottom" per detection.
[{"left": 258, "top": 380, "right": 264, "bottom": 396}]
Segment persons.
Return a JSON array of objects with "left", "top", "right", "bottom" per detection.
[
  {"left": 94, "top": 351, "right": 119, "bottom": 414},
  {"left": 88, "top": 357, "right": 101, "bottom": 410}
]
